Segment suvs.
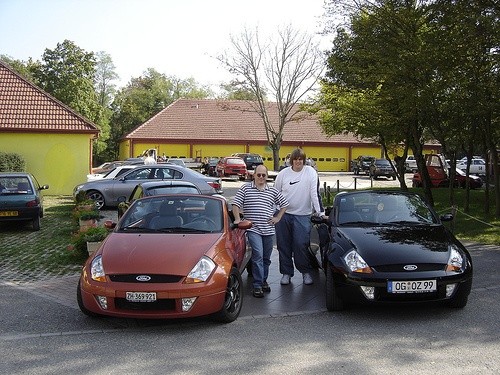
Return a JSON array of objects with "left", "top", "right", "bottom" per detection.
[
  {"left": 234, "top": 153, "right": 264, "bottom": 169},
  {"left": 370, "top": 159, "right": 397, "bottom": 181}
]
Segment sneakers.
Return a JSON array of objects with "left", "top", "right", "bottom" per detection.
[
  {"left": 302, "top": 273, "right": 312, "bottom": 285},
  {"left": 280, "top": 274, "right": 291, "bottom": 285}
]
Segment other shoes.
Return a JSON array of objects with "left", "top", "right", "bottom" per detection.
[
  {"left": 262, "top": 282, "right": 270, "bottom": 292},
  {"left": 253, "top": 288, "right": 264, "bottom": 298}
]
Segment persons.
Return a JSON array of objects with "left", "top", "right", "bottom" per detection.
[
  {"left": 202, "top": 157, "right": 209, "bottom": 166},
  {"left": 274, "top": 149, "right": 320, "bottom": 284},
  {"left": 232, "top": 164, "right": 290, "bottom": 298},
  {"left": 157, "top": 153, "right": 166, "bottom": 162}
]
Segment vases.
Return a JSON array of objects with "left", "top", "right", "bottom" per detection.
[
  {"left": 79, "top": 218, "right": 93, "bottom": 232},
  {"left": 86, "top": 241, "right": 102, "bottom": 257}
]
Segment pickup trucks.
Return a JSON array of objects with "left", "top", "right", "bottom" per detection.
[{"left": 353, "top": 155, "right": 376, "bottom": 175}]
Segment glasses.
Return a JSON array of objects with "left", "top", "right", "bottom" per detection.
[{"left": 255, "top": 173, "right": 267, "bottom": 178}]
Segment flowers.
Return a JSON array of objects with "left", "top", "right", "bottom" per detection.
[{"left": 65, "top": 199, "right": 110, "bottom": 260}]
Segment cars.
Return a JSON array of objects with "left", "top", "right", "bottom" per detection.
[
  {"left": 285, "top": 153, "right": 318, "bottom": 173},
  {"left": 73, "top": 164, "right": 224, "bottom": 212},
  {"left": 77, "top": 194, "right": 253, "bottom": 323},
  {"left": 215, "top": 156, "right": 247, "bottom": 181},
  {"left": 403, "top": 153, "right": 486, "bottom": 190},
  {"left": 127, "top": 180, "right": 205, "bottom": 226},
  {"left": 86, "top": 149, "right": 220, "bottom": 182},
  {"left": 0, "top": 171, "right": 49, "bottom": 231},
  {"left": 310, "top": 187, "right": 473, "bottom": 313}
]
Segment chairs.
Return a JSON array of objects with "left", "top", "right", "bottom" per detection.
[
  {"left": 17, "top": 182, "right": 30, "bottom": 190},
  {"left": 149, "top": 201, "right": 183, "bottom": 231},
  {"left": 339, "top": 198, "right": 362, "bottom": 223},
  {"left": 202, "top": 200, "right": 224, "bottom": 231},
  {"left": 374, "top": 195, "right": 403, "bottom": 224}
]
